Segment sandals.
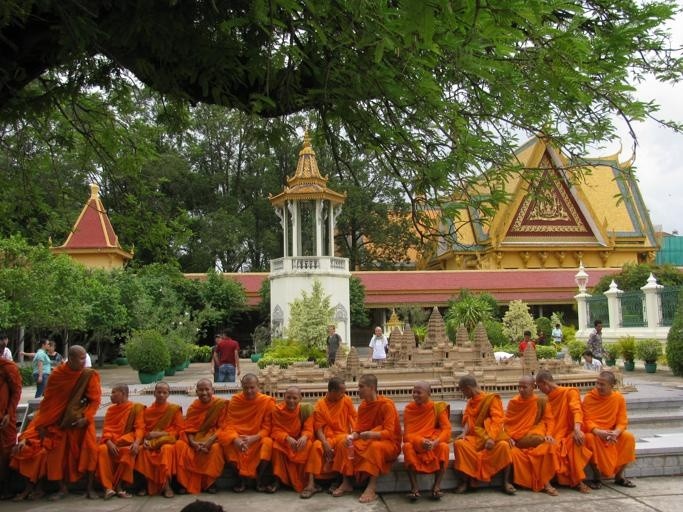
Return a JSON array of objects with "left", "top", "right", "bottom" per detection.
[
  {"left": 328, "top": 481, "right": 338, "bottom": 492},
  {"left": 410, "top": 490, "right": 427, "bottom": 502},
  {"left": 266, "top": 484, "right": 279, "bottom": 493},
  {"left": 104, "top": 489, "right": 115, "bottom": 500},
  {"left": 312, "top": 484, "right": 323, "bottom": 493},
  {"left": 503, "top": 481, "right": 515, "bottom": 500},
  {"left": 255, "top": 484, "right": 265, "bottom": 490},
  {"left": 208, "top": 485, "right": 218, "bottom": 494},
  {"left": 233, "top": 484, "right": 245, "bottom": 492},
  {"left": 333, "top": 486, "right": 355, "bottom": 496},
  {"left": 29, "top": 490, "right": 44, "bottom": 499},
  {"left": 177, "top": 486, "right": 187, "bottom": 495},
  {"left": 117, "top": 489, "right": 132, "bottom": 497},
  {"left": 616, "top": 477, "right": 638, "bottom": 489},
  {"left": 300, "top": 488, "right": 314, "bottom": 498},
  {"left": 539, "top": 482, "right": 559, "bottom": 494},
  {"left": 434, "top": 487, "right": 442, "bottom": 500},
  {"left": 573, "top": 481, "right": 591, "bottom": 492},
  {"left": 137, "top": 486, "right": 148, "bottom": 496},
  {"left": 83, "top": 491, "right": 98, "bottom": 500},
  {"left": 163, "top": 488, "right": 173, "bottom": 498},
  {"left": 468, "top": 479, "right": 479, "bottom": 488},
  {"left": 49, "top": 491, "right": 68, "bottom": 500},
  {"left": 14, "top": 490, "right": 28, "bottom": 500},
  {"left": 585, "top": 474, "right": 605, "bottom": 489},
  {"left": 360, "top": 487, "right": 379, "bottom": 505},
  {"left": 455, "top": 482, "right": 468, "bottom": 493}
]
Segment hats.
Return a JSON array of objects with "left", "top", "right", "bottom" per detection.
[{"left": 214, "top": 334, "right": 222, "bottom": 337}]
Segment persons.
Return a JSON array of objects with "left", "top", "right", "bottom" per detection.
[
  {"left": 538, "top": 331, "right": 546, "bottom": 344},
  {"left": 32, "top": 338, "right": 51, "bottom": 398},
  {"left": 97, "top": 383, "right": 147, "bottom": 500},
  {"left": 37, "top": 343, "right": 103, "bottom": 503},
  {"left": 12, "top": 395, "right": 89, "bottom": 501},
  {"left": 587, "top": 320, "right": 608, "bottom": 358},
  {"left": 19, "top": 340, "right": 63, "bottom": 372},
  {"left": 451, "top": 375, "right": 517, "bottom": 495},
  {"left": 332, "top": 373, "right": 403, "bottom": 504},
  {"left": 326, "top": 325, "right": 342, "bottom": 367},
  {"left": 581, "top": 350, "right": 602, "bottom": 373},
  {"left": 0, "top": 341, "right": 23, "bottom": 500},
  {"left": 551, "top": 324, "right": 563, "bottom": 343},
  {"left": 61, "top": 351, "right": 92, "bottom": 368},
  {"left": 581, "top": 371, "right": 637, "bottom": 489},
  {"left": 210, "top": 334, "right": 230, "bottom": 382},
  {"left": 300, "top": 376, "right": 358, "bottom": 499},
  {"left": 534, "top": 369, "right": 594, "bottom": 494},
  {"left": 0, "top": 334, "right": 14, "bottom": 361},
  {"left": 518, "top": 330, "right": 536, "bottom": 352},
  {"left": 368, "top": 326, "right": 390, "bottom": 367},
  {"left": 219, "top": 373, "right": 275, "bottom": 492},
  {"left": 214, "top": 327, "right": 241, "bottom": 382},
  {"left": 267, "top": 386, "right": 322, "bottom": 493},
  {"left": 136, "top": 381, "right": 186, "bottom": 498},
  {"left": 482, "top": 350, "right": 514, "bottom": 361},
  {"left": 174, "top": 378, "right": 231, "bottom": 494},
  {"left": 402, "top": 380, "right": 452, "bottom": 499},
  {"left": 505, "top": 373, "right": 561, "bottom": 497}
]
